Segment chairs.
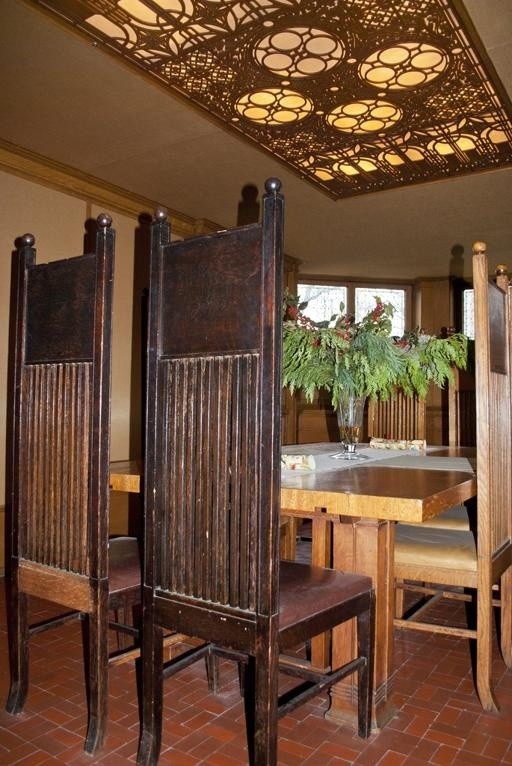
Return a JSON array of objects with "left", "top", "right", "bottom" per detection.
[
  {"left": 4, "top": 214, "right": 221, "bottom": 758},
  {"left": 135, "top": 178, "right": 377, "bottom": 766},
  {"left": 448, "top": 366, "right": 477, "bottom": 447},
  {"left": 366, "top": 379, "right": 427, "bottom": 444},
  {"left": 391, "top": 240, "right": 512, "bottom": 716}
]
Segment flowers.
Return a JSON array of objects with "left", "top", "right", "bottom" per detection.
[{"left": 280, "top": 286, "right": 470, "bottom": 443}]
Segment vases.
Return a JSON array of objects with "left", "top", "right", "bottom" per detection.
[{"left": 328, "top": 394, "right": 370, "bottom": 463}]
